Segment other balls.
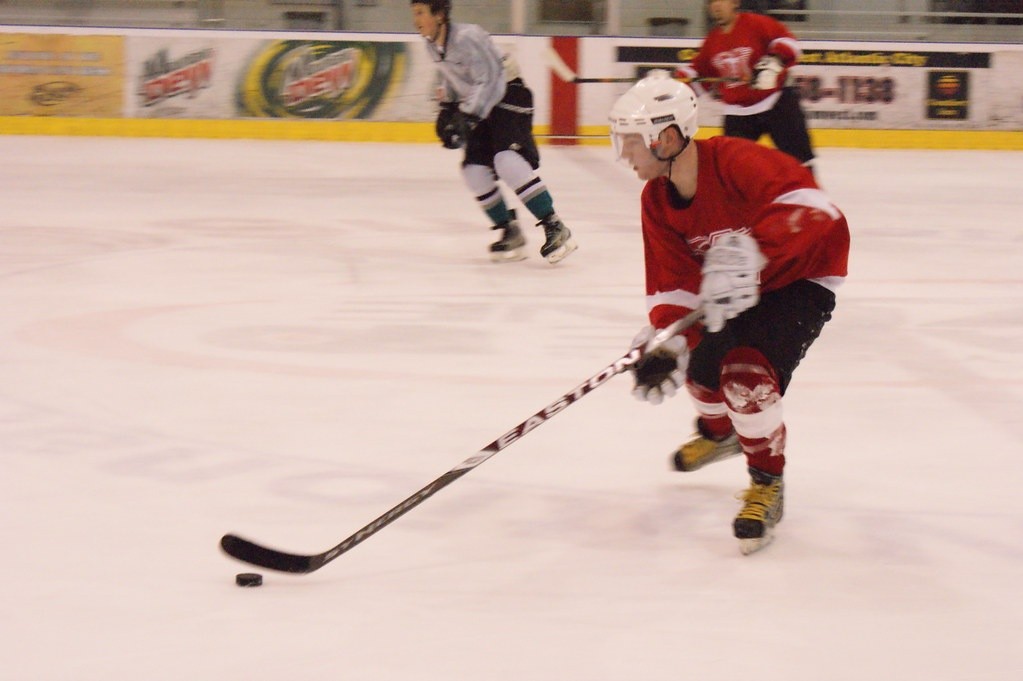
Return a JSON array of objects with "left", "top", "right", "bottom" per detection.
[{"left": 236, "top": 573, "right": 261, "bottom": 586}]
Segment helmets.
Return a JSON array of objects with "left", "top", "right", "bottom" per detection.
[
  {"left": 412, "top": 0, "right": 450, "bottom": 20},
  {"left": 609, "top": 69, "right": 699, "bottom": 154}
]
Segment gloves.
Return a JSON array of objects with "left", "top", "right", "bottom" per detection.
[
  {"left": 697, "top": 233, "right": 760, "bottom": 332},
  {"left": 434, "top": 103, "right": 462, "bottom": 141},
  {"left": 442, "top": 111, "right": 483, "bottom": 149},
  {"left": 752, "top": 53, "right": 787, "bottom": 90},
  {"left": 627, "top": 329, "right": 692, "bottom": 401}
]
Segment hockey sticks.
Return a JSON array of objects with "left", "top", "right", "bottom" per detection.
[
  {"left": 537, "top": 47, "right": 805, "bottom": 87},
  {"left": 221, "top": 311, "right": 716, "bottom": 577}
]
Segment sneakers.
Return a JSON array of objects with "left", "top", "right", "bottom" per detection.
[
  {"left": 537, "top": 214, "right": 577, "bottom": 265},
  {"left": 488, "top": 210, "right": 528, "bottom": 264},
  {"left": 732, "top": 465, "right": 785, "bottom": 557},
  {"left": 671, "top": 417, "right": 740, "bottom": 473}
]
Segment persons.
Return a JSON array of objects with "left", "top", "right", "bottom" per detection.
[
  {"left": 411, "top": 0, "right": 579, "bottom": 263},
  {"left": 606, "top": 71, "right": 851, "bottom": 555},
  {"left": 673, "top": 0, "right": 815, "bottom": 174}
]
